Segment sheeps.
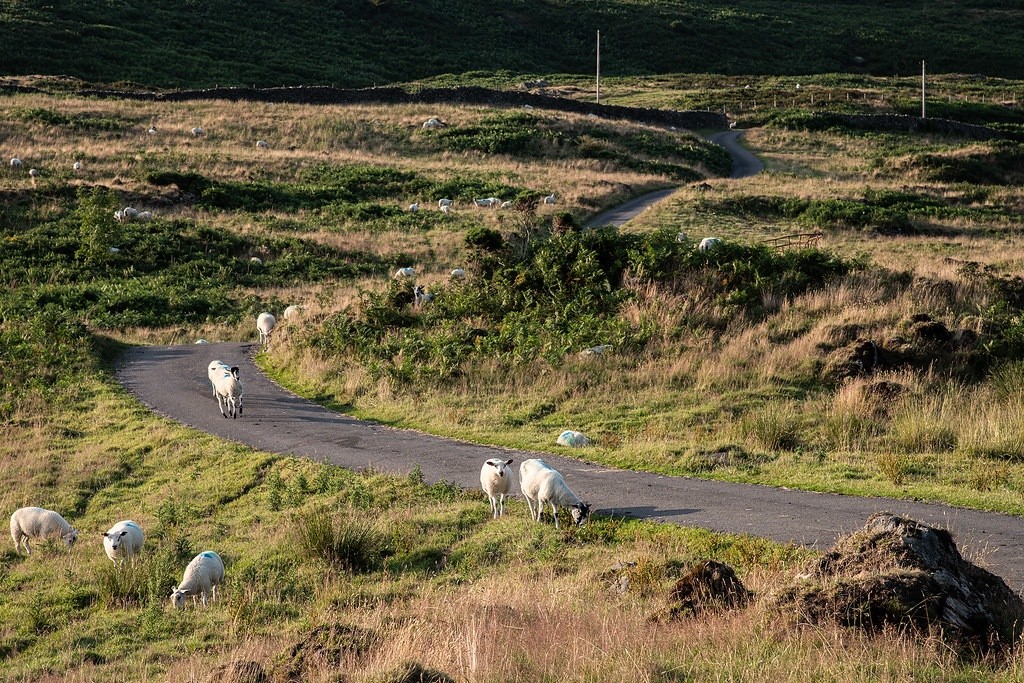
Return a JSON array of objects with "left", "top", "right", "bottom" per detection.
[
  {"left": 147, "top": 125, "right": 159, "bottom": 136},
  {"left": 9, "top": 506, "right": 79, "bottom": 557},
  {"left": 410, "top": 284, "right": 439, "bottom": 308},
  {"left": 113, "top": 205, "right": 153, "bottom": 223},
  {"left": 668, "top": 230, "right": 723, "bottom": 256},
  {"left": 472, "top": 196, "right": 514, "bottom": 210},
  {"left": 393, "top": 267, "right": 417, "bottom": 279},
  {"left": 190, "top": 127, "right": 204, "bottom": 136},
  {"left": 255, "top": 140, "right": 271, "bottom": 150},
  {"left": 256, "top": 312, "right": 277, "bottom": 353},
  {"left": 479, "top": 457, "right": 514, "bottom": 521},
  {"left": 543, "top": 193, "right": 558, "bottom": 205},
  {"left": 437, "top": 198, "right": 455, "bottom": 214},
  {"left": 250, "top": 256, "right": 262, "bottom": 267},
  {"left": 588, "top": 113, "right": 599, "bottom": 119},
  {"left": 168, "top": 549, "right": 225, "bottom": 613},
  {"left": 525, "top": 104, "right": 533, "bottom": 110},
  {"left": 518, "top": 458, "right": 593, "bottom": 530},
  {"left": 422, "top": 118, "right": 440, "bottom": 129},
  {"left": 408, "top": 202, "right": 421, "bottom": 212},
  {"left": 670, "top": 127, "right": 676, "bottom": 130},
  {"left": 556, "top": 429, "right": 590, "bottom": 448},
  {"left": 207, "top": 359, "right": 244, "bottom": 420},
  {"left": 72, "top": 161, "right": 80, "bottom": 170},
  {"left": 100, "top": 519, "right": 146, "bottom": 571},
  {"left": 451, "top": 268, "right": 466, "bottom": 279},
  {"left": 194, "top": 339, "right": 207, "bottom": 344},
  {"left": 730, "top": 121, "right": 737, "bottom": 130},
  {"left": 283, "top": 304, "right": 307, "bottom": 319},
  {"left": 28, "top": 168, "right": 38, "bottom": 177},
  {"left": 10, "top": 158, "right": 22, "bottom": 166}
]
